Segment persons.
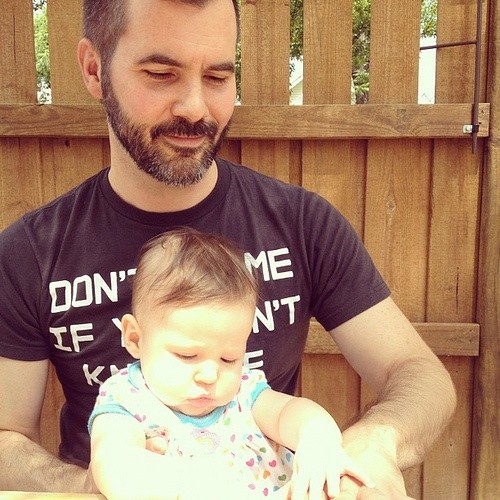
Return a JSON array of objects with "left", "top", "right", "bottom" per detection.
[
  {"left": 1, "top": 0, "right": 460, "bottom": 500},
  {"left": 84, "top": 227, "right": 347, "bottom": 500}
]
